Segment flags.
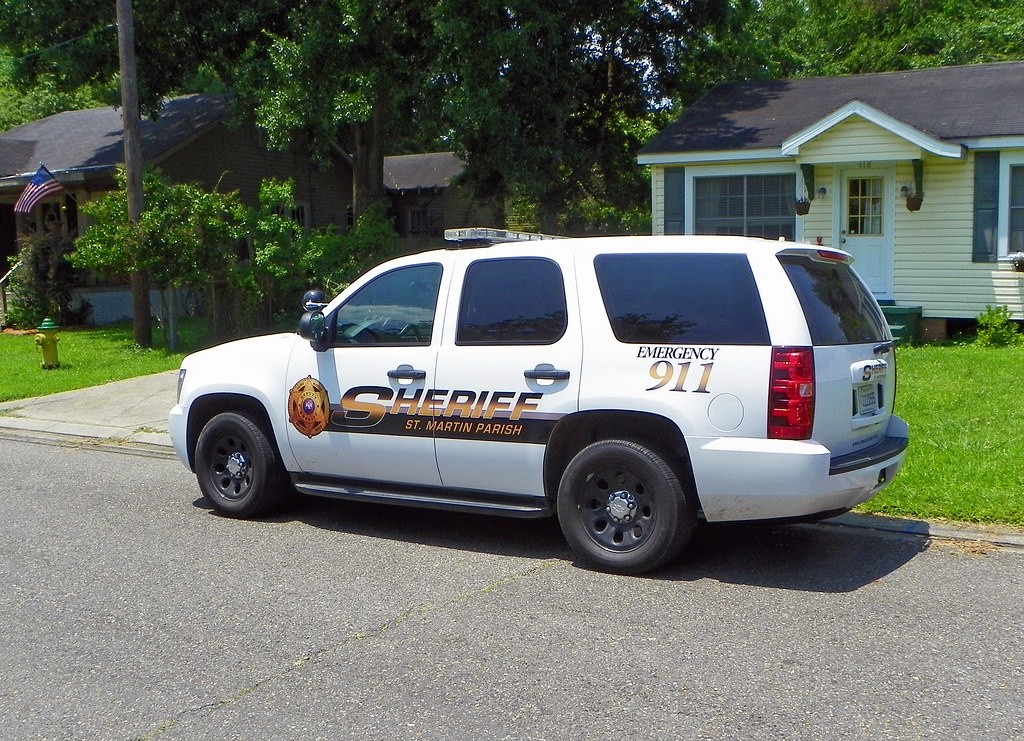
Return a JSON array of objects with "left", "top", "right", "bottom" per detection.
[{"left": 15, "top": 164, "right": 63, "bottom": 214}]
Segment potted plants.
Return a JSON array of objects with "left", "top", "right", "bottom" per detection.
[
  {"left": 902, "top": 188, "right": 925, "bottom": 211},
  {"left": 795, "top": 196, "right": 810, "bottom": 215}
]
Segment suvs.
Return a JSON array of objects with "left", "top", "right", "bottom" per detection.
[{"left": 167, "top": 227, "right": 910, "bottom": 575}]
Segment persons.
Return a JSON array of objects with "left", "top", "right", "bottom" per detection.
[{"left": 33, "top": 215, "right": 75, "bottom": 313}]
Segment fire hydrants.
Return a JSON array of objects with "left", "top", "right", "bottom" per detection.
[{"left": 34, "top": 315, "right": 61, "bottom": 369}]
